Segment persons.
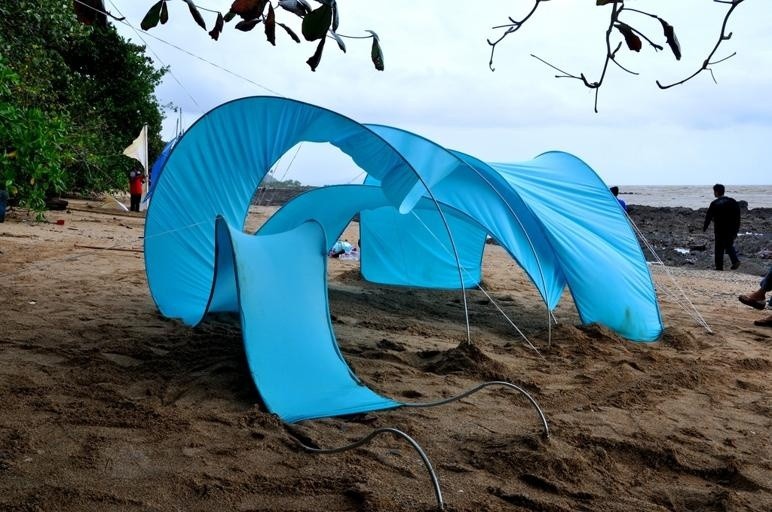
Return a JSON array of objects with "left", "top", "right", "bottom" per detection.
[
  {"left": 702, "top": 183, "right": 741, "bottom": 270},
  {"left": 610, "top": 185, "right": 626, "bottom": 211},
  {"left": 128, "top": 171, "right": 149, "bottom": 212},
  {"left": 738, "top": 266, "right": 772, "bottom": 327},
  {"left": 127, "top": 166, "right": 140, "bottom": 183}
]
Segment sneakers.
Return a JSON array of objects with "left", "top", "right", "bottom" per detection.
[
  {"left": 738, "top": 295, "right": 766, "bottom": 311},
  {"left": 754, "top": 314, "right": 772, "bottom": 326}
]
